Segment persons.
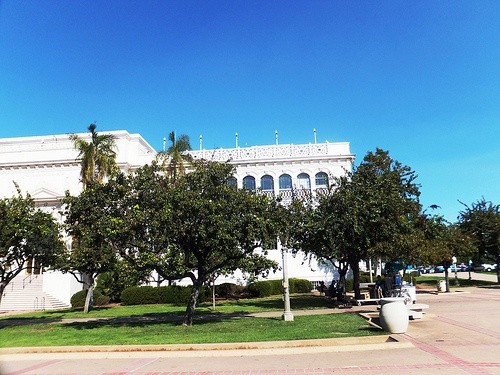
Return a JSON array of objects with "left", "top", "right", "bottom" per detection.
[
  {"left": 329, "top": 280, "right": 341, "bottom": 302},
  {"left": 392, "top": 273, "right": 401, "bottom": 286},
  {"left": 377, "top": 274, "right": 392, "bottom": 296}
]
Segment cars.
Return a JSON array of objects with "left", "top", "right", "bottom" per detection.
[{"left": 415, "top": 263, "right": 495, "bottom": 274}]
